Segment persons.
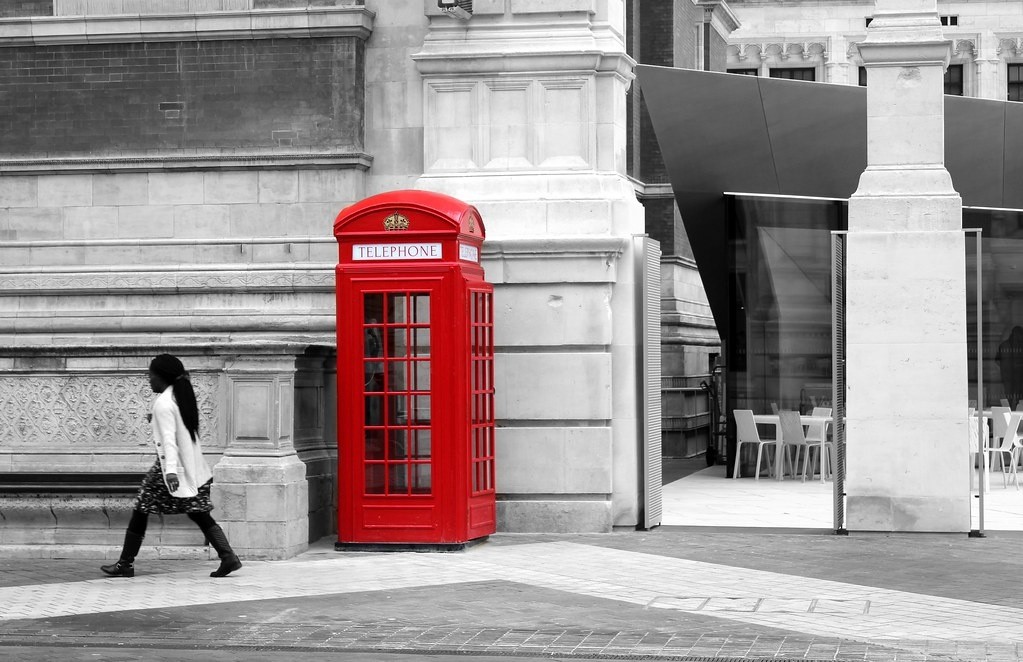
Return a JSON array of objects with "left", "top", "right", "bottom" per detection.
[{"left": 101, "top": 354, "right": 242, "bottom": 577}]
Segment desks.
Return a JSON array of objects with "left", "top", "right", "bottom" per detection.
[
  {"left": 751, "top": 414, "right": 845, "bottom": 480},
  {"left": 974, "top": 409, "right": 1023, "bottom": 471}
]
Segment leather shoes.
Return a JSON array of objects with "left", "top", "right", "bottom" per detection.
[
  {"left": 100, "top": 560, "right": 134, "bottom": 577},
  {"left": 210, "top": 556, "right": 242, "bottom": 576}
]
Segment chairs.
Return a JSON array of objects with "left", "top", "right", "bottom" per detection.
[{"left": 731, "top": 399, "right": 1023, "bottom": 491}]
